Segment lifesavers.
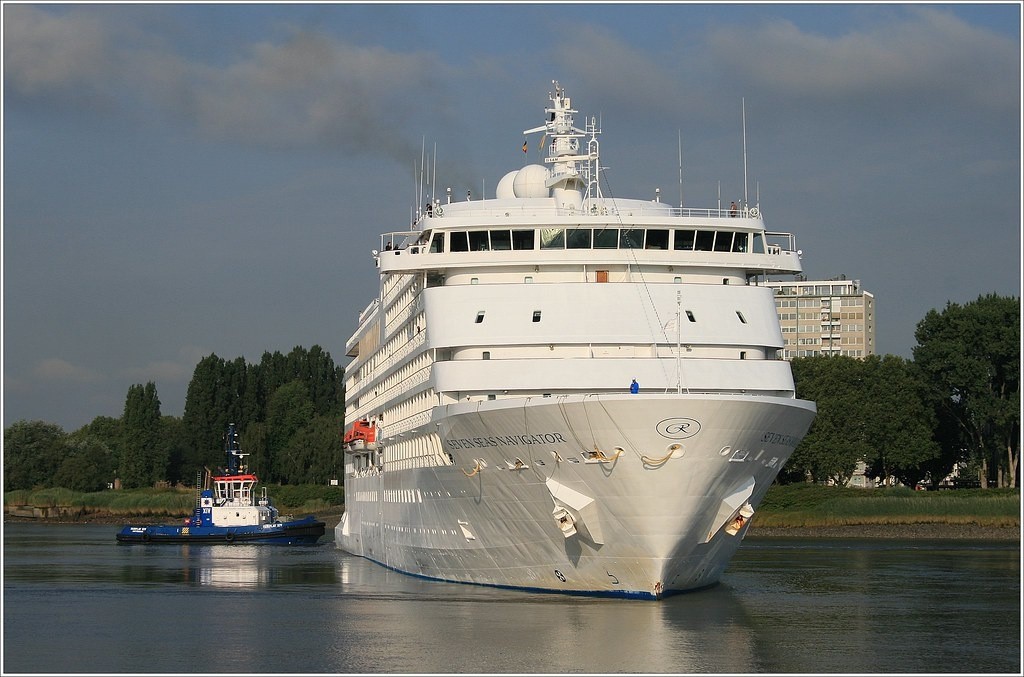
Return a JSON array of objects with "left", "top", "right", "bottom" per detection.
[
  {"left": 184, "top": 518, "right": 191, "bottom": 525},
  {"left": 225, "top": 533, "right": 234, "bottom": 541}
]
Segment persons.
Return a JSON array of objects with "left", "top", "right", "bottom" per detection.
[
  {"left": 426, "top": 203, "right": 432, "bottom": 218},
  {"left": 386, "top": 242, "right": 399, "bottom": 251},
  {"left": 730, "top": 201, "right": 737, "bottom": 218},
  {"left": 424, "top": 237, "right": 428, "bottom": 245}
]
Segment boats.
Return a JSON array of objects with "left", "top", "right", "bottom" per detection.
[
  {"left": 342, "top": 419, "right": 377, "bottom": 456},
  {"left": 115, "top": 418, "right": 327, "bottom": 546}
]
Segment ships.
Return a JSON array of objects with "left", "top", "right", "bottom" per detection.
[{"left": 333, "top": 75, "right": 819, "bottom": 601}]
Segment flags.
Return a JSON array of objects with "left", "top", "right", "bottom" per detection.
[{"left": 521, "top": 140, "right": 527, "bottom": 153}]
